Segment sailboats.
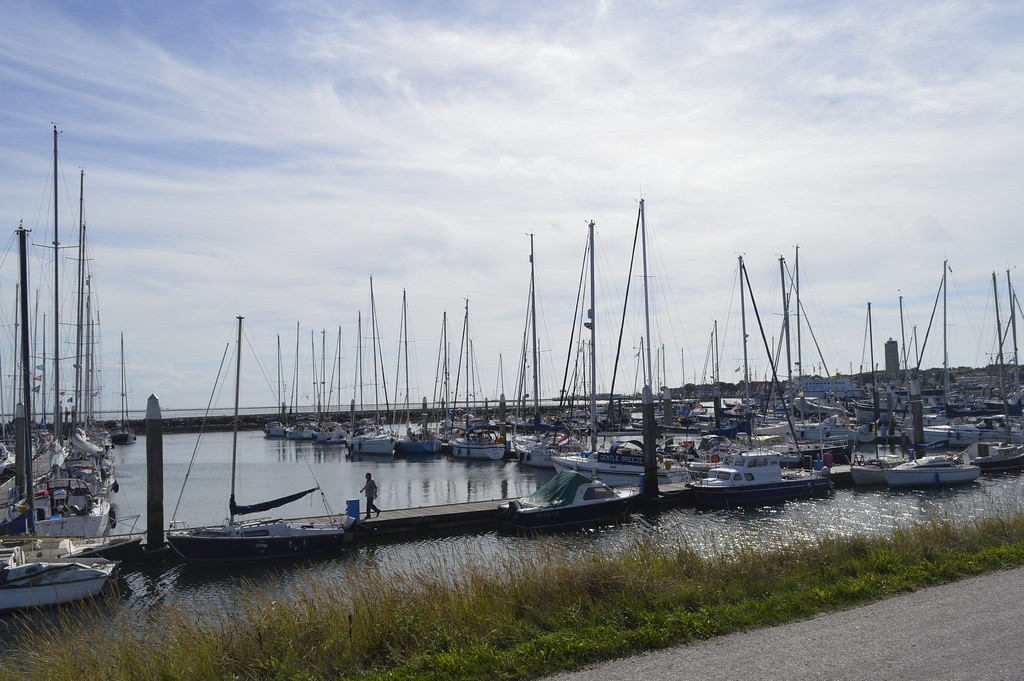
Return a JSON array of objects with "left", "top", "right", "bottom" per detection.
[
  {"left": 260, "top": 196, "right": 1024, "bottom": 494},
  {"left": 166, "top": 312, "right": 357, "bottom": 566},
  {"left": 0, "top": 121, "right": 141, "bottom": 617}
]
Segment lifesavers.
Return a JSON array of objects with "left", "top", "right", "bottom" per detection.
[
  {"left": 109, "top": 507, "right": 116, "bottom": 529},
  {"left": 710, "top": 452, "right": 720, "bottom": 464}
]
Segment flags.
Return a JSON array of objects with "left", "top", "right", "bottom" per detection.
[
  {"left": 35, "top": 364, "right": 44, "bottom": 370},
  {"left": 558, "top": 435, "right": 570, "bottom": 448},
  {"left": 67, "top": 397, "right": 72, "bottom": 403},
  {"left": 31, "top": 385, "right": 40, "bottom": 394},
  {"left": 33, "top": 375, "right": 42, "bottom": 381}
]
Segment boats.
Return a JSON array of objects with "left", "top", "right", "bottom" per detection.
[
  {"left": 684, "top": 414, "right": 835, "bottom": 514},
  {"left": 495, "top": 466, "right": 642, "bottom": 541}
]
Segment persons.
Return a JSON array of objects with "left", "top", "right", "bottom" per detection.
[
  {"left": 66, "top": 494, "right": 93, "bottom": 516},
  {"left": 498, "top": 434, "right": 505, "bottom": 444},
  {"left": 900, "top": 431, "right": 910, "bottom": 458},
  {"left": 360, "top": 473, "right": 381, "bottom": 519}
]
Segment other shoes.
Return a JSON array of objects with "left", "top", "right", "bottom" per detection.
[
  {"left": 364, "top": 515, "right": 371, "bottom": 520},
  {"left": 376, "top": 510, "right": 380, "bottom": 517}
]
